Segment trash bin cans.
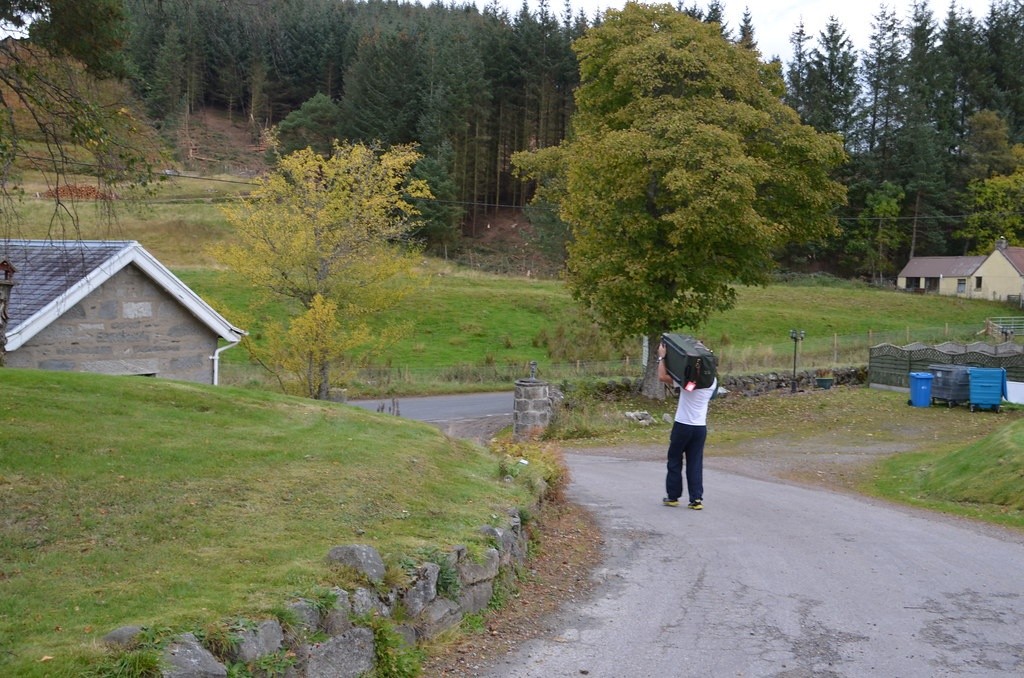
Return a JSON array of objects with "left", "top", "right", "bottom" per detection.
[
  {"left": 908, "top": 372, "right": 934, "bottom": 407},
  {"left": 966, "top": 366, "right": 1008, "bottom": 413},
  {"left": 926, "top": 363, "right": 970, "bottom": 409}
]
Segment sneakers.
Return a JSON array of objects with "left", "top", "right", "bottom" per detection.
[
  {"left": 663, "top": 498, "right": 679, "bottom": 506},
  {"left": 687, "top": 501, "right": 704, "bottom": 510}
]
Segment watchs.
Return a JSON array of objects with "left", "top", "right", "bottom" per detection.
[{"left": 658, "top": 356, "right": 665, "bottom": 361}]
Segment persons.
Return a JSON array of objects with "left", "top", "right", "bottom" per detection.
[{"left": 656, "top": 340, "right": 718, "bottom": 510}]
